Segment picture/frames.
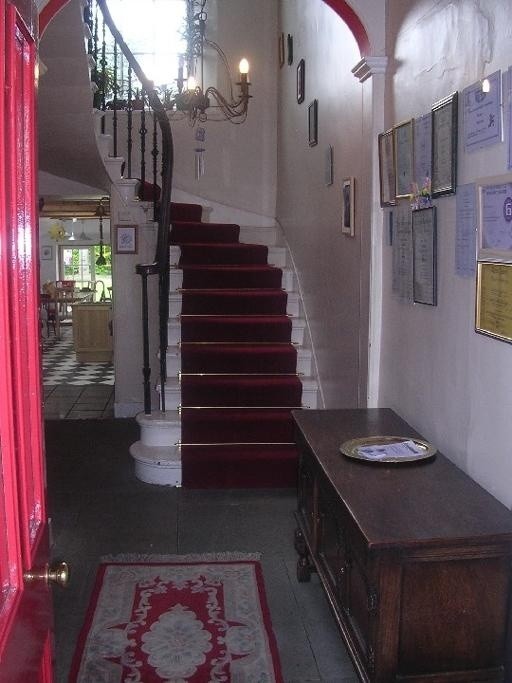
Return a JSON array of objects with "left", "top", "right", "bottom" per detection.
[
  {"left": 114, "top": 225, "right": 138, "bottom": 255},
  {"left": 378, "top": 65, "right": 512, "bottom": 344},
  {"left": 277, "top": 31, "right": 355, "bottom": 237},
  {"left": 40, "top": 245, "right": 52, "bottom": 260}
]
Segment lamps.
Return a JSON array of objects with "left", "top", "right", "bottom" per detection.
[
  {"left": 64, "top": 222, "right": 79, "bottom": 241},
  {"left": 95, "top": 197, "right": 109, "bottom": 265},
  {"left": 142, "top": 0, "right": 253, "bottom": 180}
]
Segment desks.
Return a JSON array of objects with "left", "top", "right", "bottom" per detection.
[{"left": 49, "top": 291, "right": 97, "bottom": 337}]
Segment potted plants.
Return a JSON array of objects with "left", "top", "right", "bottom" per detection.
[{"left": 91, "top": 62, "right": 197, "bottom": 110}]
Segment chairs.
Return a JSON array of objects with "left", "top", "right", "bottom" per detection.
[{"left": 41, "top": 294, "right": 56, "bottom": 336}]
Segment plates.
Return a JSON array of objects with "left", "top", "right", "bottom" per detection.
[{"left": 339, "top": 435, "right": 437, "bottom": 462}]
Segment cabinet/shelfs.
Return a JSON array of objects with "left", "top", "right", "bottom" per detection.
[
  {"left": 70, "top": 303, "right": 114, "bottom": 361},
  {"left": 289, "top": 407, "right": 512, "bottom": 683}
]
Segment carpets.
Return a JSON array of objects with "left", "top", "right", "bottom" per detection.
[{"left": 68, "top": 551, "right": 285, "bottom": 683}]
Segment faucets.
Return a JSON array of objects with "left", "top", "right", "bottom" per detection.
[{"left": 92, "top": 279, "right": 105, "bottom": 302}]
